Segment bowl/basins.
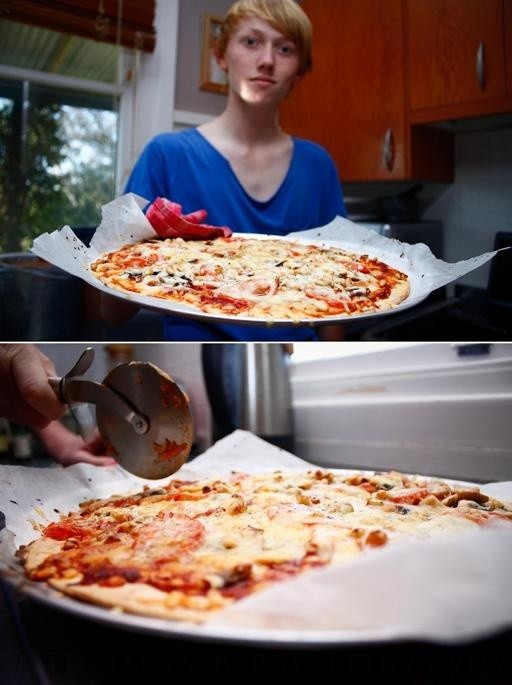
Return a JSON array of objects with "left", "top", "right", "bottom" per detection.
[{"left": 0, "top": 252, "right": 139, "bottom": 341}]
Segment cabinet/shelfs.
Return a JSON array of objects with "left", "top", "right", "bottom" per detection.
[
  {"left": 277, "top": 0, "right": 456, "bottom": 182},
  {"left": 409, "top": 0, "right": 512, "bottom": 125}
]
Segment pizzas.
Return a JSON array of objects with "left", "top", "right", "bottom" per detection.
[{"left": 15, "top": 467, "right": 512, "bottom": 623}]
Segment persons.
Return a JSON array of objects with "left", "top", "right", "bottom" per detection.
[
  {"left": 123, "top": 1, "right": 348, "bottom": 341},
  {"left": 0, "top": 345, "right": 117, "bottom": 466}
]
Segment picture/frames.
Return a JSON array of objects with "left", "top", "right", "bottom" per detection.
[{"left": 198, "top": 12, "right": 230, "bottom": 95}]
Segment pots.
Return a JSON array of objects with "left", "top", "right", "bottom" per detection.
[{"left": 374, "top": 184, "right": 424, "bottom": 224}]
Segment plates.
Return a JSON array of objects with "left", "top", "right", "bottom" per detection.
[{"left": 1, "top": 527, "right": 512, "bottom": 646}]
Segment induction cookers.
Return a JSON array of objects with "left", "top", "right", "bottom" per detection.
[{"left": 368, "top": 291, "right": 511, "bottom": 340}]
